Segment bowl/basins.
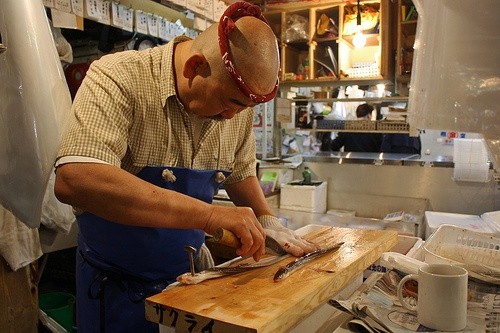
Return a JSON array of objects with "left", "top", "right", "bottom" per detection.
[{"left": 314, "top": 91, "right": 327, "bottom": 99}]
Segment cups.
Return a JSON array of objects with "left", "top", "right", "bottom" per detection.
[
  {"left": 397, "top": 263, "right": 468, "bottom": 331},
  {"left": 285, "top": 73, "right": 294, "bottom": 81}
]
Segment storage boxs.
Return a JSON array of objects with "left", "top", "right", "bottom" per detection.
[{"left": 161, "top": 179, "right": 500, "bottom": 333}]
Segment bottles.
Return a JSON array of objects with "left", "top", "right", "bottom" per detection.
[
  {"left": 298, "top": 107, "right": 307, "bottom": 127},
  {"left": 296, "top": 60, "right": 309, "bottom": 80},
  {"left": 302, "top": 166, "right": 311, "bottom": 184}
]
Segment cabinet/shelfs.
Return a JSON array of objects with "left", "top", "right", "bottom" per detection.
[{"left": 282, "top": 0, "right": 420, "bottom": 86}]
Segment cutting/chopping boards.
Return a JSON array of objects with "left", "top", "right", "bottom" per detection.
[{"left": 144, "top": 223, "right": 399, "bottom": 333}]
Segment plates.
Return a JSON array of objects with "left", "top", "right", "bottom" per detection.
[
  {"left": 292, "top": 96, "right": 312, "bottom": 99},
  {"left": 453, "top": 136, "right": 491, "bottom": 182}
]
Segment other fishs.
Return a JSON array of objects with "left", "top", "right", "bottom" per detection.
[
  {"left": 176, "top": 253, "right": 292, "bottom": 284},
  {"left": 273, "top": 242, "right": 345, "bottom": 282}
]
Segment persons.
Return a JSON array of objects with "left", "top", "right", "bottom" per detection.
[
  {"left": 330, "top": 104, "right": 380, "bottom": 154},
  {"left": 54, "top": 3, "right": 316, "bottom": 333}
]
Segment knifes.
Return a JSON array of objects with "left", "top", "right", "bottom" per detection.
[{"left": 212, "top": 226, "right": 287, "bottom": 257}]
